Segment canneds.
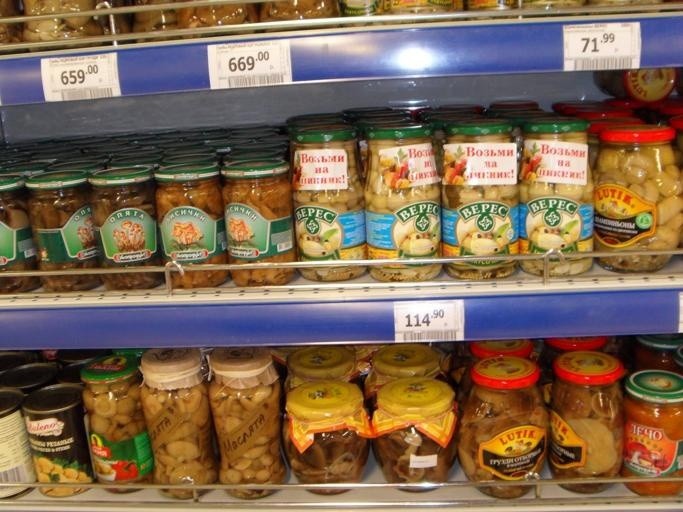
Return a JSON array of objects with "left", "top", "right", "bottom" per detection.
[
  {"left": 1, "top": 125, "right": 282, "bottom": 169},
  {"left": 79, "top": 354, "right": 152, "bottom": 495},
  {"left": 27, "top": 172, "right": 100, "bottom": 292},
  {"left": 286, "top": 99, "right": 557, "bottom": 122},
  {"left": 368, "top": 378, "right": 460, "bottom": 494},
  {"left": 282, "top": 382, "right": 370, "bottom": 494},
  {"left": 543, "top": 337, "right": 606, "bottom": 395},
  {"left": 22, "top": 388, "right": 94, "bottom": 499},
  {"left": 592, "top": 130, "right": 683, "bottom": 273},
  {"left": 220, "top": 160, "right": 297, "bottom": 286},
  {"left": 140, "top": 348, "right": 218, "bottom": 499},
  {"left": 365, "top": 126, "right": 440, "bottom": 280},
  {"left": 459, "top": 356, "right": 554, "bottom": 498},
  {"left": 672, "top": 342, "right": 682, "bottom": 371},
  {"left": 0, "top": 0, "right": 675, "bottom": 52},
  {"left": 0, "top": 349, "right": 84, "bottom": 388},
  {"left": 90, "top": 168, "right": 161, "bottom": 289},
  {"left": 518, "top": 116, "right": 595, "bottom": 274},
  {"left": 155, "top": 165, "right": 230, "bottom": 287},
  {"left": 587, "top": 68, "right": 677, "bottom": 99},
  {"left": 468, "top": 340, "right": 536, "bottom": 359},
  {"left": 289, "top": 347, "right": 360, "bottom": 386},
  {"left": 0, "top": 178, "right": 39, "bottom": 290},
  {"left": 290, "top": 129, "right": 366, "bottom": 281},
  {"left": 0, "top": 390, "right": 38, "bottom": 503},
  {"left": 364, "top": 345, "right": 450, "bottom": 405},
  {"left": 544, "top": 352, "right": 623, "bottom": 491},
  {"left": 438, "top": 117, "right": 519, "bottom": 280},
  {"left": 207, "top": 346, "right": 287, "bottom": 499},
  {"left": 551, "top": 100, "right": 683, "bottom": 127}
]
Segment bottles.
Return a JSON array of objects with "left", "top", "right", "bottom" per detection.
[
  {"left": 673, "top": 341, "right": 682, "bottom": 376},
  {"left": 79, "top": 348, "right": 287, "bottom": 500}
]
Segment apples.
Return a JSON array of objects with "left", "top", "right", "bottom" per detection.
[{"left": 111, "top": 460, "right": 137, "bottom": 483}]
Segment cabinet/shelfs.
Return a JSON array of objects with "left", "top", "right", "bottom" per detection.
[{"left": 0, "top": 0, "right": 682, "bottom": 509}]
